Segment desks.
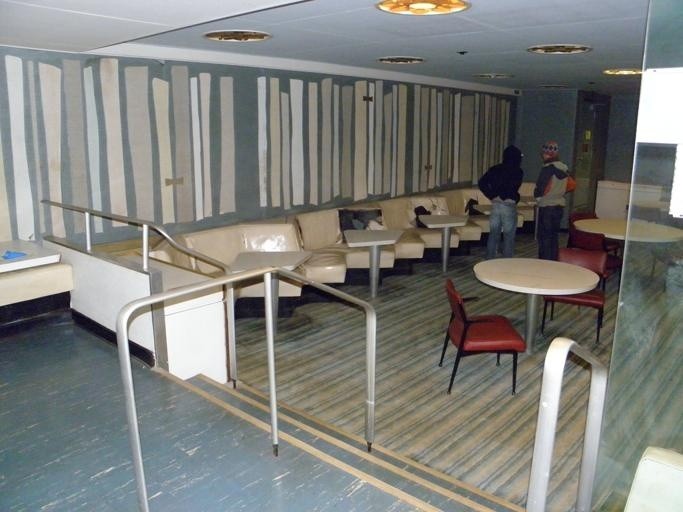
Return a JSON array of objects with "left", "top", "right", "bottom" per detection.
[
  {"left": 594, "top": 180, "right": 667, "bottom": 219},
  {"left": 473, "top": 257, "right": 599, "bottom": 356}
]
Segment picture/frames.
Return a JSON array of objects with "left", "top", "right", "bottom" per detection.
[{"left": 594, "top": 180, "right": 667, "bottom": 219}]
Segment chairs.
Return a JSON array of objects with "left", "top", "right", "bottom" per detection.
[
  {"left": 568, "top": 206, "right": 682, "bottom": 292},
  {"left": 541, "top": 247, "right": 608, "bottom": 344},
  {"left": 439, "top": 279, "right": 526, "bottom": 394}
]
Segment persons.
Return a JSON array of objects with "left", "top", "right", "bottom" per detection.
[
  {"left": 477, "top": 144, "right": 524, "bottom": 260},
  {"left": 532, "top": 139, "right": 577, "bottom": 262}
]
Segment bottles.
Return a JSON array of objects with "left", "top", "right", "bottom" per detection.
[{"left": 541, "top": 140, "right": 560, "bottom": 157}]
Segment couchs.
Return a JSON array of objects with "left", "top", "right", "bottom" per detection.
[{"left": 150, "top": 182, "right": 537, "bottom": 316}]
